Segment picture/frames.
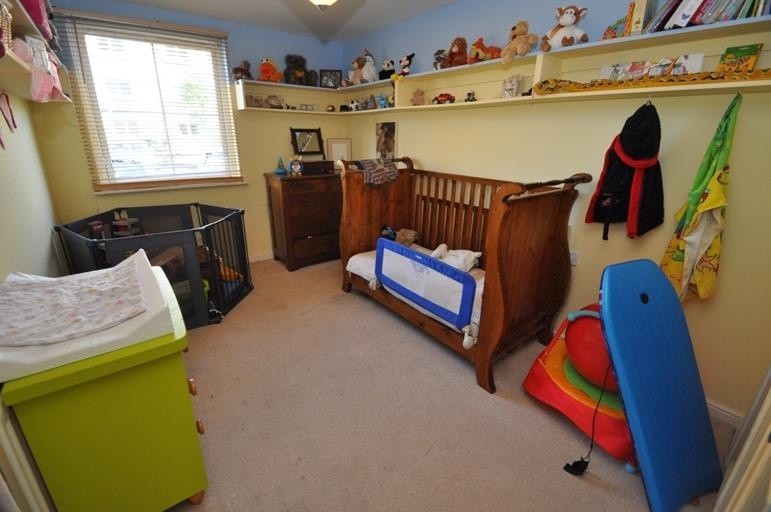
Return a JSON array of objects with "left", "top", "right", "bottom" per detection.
[
  {"left": 375, "top": 122, "right": 399, "bottom": 159},
  {"left": 320, "top": 69, "right": 342, "bottom": 89},
  {"left": 290, "top": 128, "right": 324, "bottom": 155},
  {"left": 327, "top": 137, "right": 352, "bottom": 170}
]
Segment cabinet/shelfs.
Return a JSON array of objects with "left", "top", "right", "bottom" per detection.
[
  {"left": 233, "top": 15, "right": 771, "bottom": 115},
  {"left": 263, "top": 167, "right": 341, "bottom": 272},
  {"left": 0, "top": 266, "right": 210, "bottom": 512},
  {"left": 0, "top": 0, "right": 73, "bottom": 103}
]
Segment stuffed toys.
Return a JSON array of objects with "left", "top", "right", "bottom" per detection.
[
  {"left": 380, "top": 225, "right": 419, "bottom": 249},
  {"left": 231, "top": 5, "right": 589, "bottom": 110}
]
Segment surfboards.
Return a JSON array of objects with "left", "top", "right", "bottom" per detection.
[{"left": 599, "top": 257, "right": 725, "bottom": 512}]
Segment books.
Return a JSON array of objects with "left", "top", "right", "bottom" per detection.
[{"left": 632, "top": 0, "right": 771, "bottom": 36}]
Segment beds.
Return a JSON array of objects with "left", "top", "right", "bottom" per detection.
[{"left": 338, "top": 156, "right": 593, "bottom": 395}]
